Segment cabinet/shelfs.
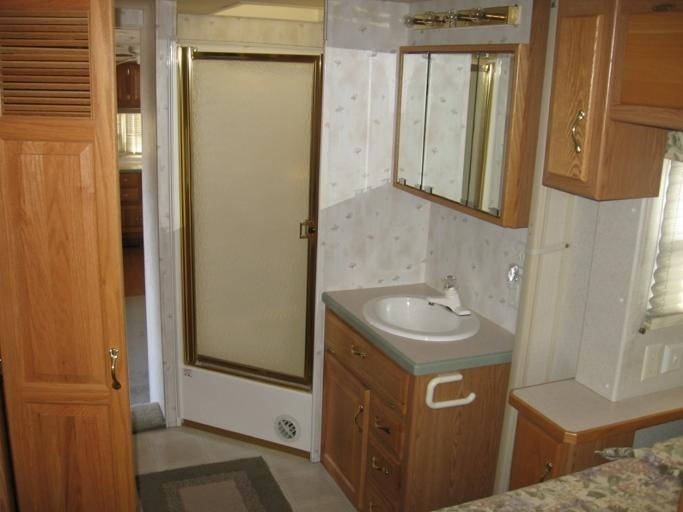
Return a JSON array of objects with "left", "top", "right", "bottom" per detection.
[
  {"left": 542, "top": 0, "right": 682, "bottom": 202},
  {"left": 512, "top": 414, "right": 633, "bottom": 490},
  {"left": 321, "top": 305, "right": 511, "bottom": 512}
]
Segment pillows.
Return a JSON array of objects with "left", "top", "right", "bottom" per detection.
[{"left": 393, "top": 43, "right": 530, "bottom": 228}]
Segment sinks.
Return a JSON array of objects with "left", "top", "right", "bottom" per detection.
[{"left": 363, "top": 293, "right": 480, "bottom": 340}]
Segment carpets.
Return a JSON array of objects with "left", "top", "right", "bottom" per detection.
[
  {"left": 125, "top": 294, "right": 166, "bottom": 433},
  {"left": 135, "top": 456, "right": 294, "bottom": 512}
]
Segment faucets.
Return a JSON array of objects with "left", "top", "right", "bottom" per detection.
[{"left": 426, "top": 275, "right": 472, "bottom": 316}]
